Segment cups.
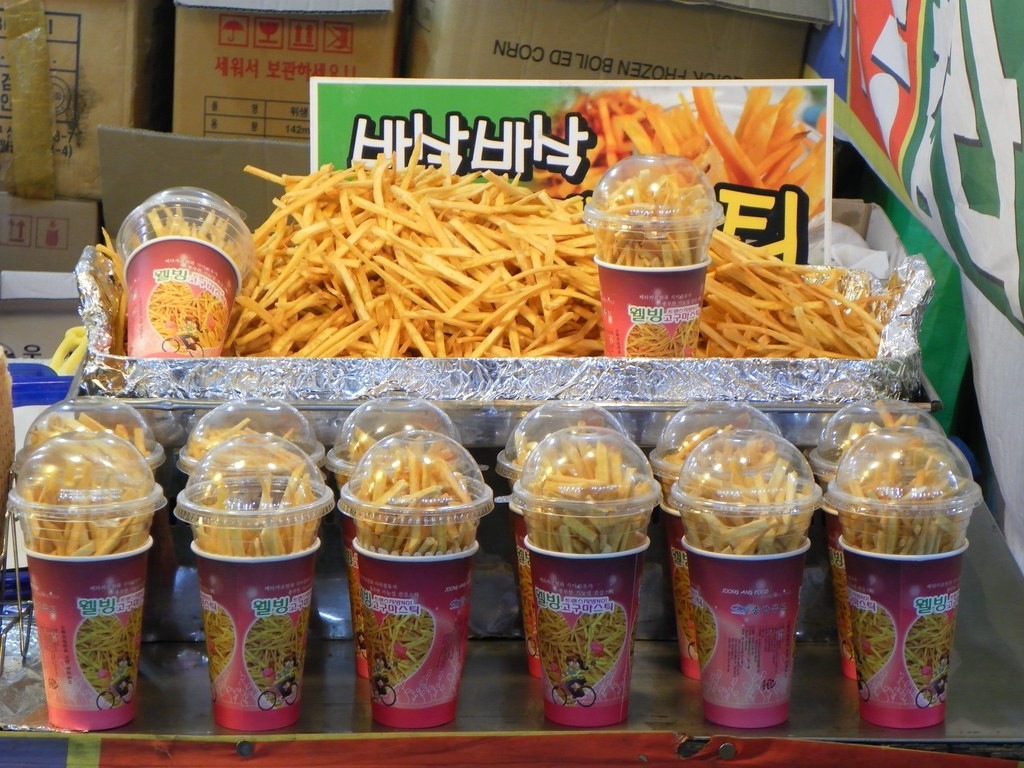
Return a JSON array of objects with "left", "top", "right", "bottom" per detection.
[
  {"left": 115, "top": 182, "right": 255, "bottom": 360},
  {"left": 584, "top": 153, "right": 724, "bottom": 353},
  {"left": 11, "top": 394, "right": 985, "bottom": 734}
]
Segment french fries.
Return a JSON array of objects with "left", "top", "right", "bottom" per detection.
[
  {"left": 16, "top": 402, "right": 977, "bottom": 557},
  {"left": 534, "top": 86, "right": 828, "bottom": 223},
  {"left": 97, "top": 129, "right": 901, "bottom": 363}
]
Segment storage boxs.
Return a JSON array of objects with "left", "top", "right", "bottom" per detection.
[
  {"left": 403, "top": 0, "right": 835, "bottom": 80},
  {"left": 0, "top": 193, "right": 99, "bottom": 273},
  {"left": 0, "top": 0, "right": 172, "bottom": 201},
  {"left": 173, "top": 0, "right": 403, "bottom": 142}
]
